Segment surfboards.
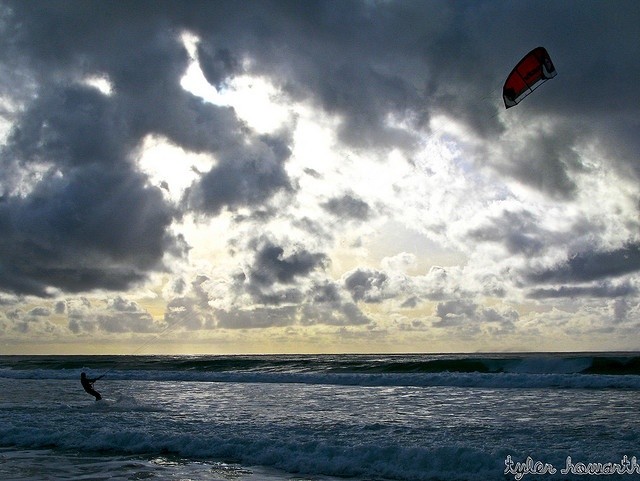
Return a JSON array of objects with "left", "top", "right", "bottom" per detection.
[{"left": 100, "top": 398, "right": 116, "bottom": 403}]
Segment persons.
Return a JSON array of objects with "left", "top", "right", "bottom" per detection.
[{"left": 81, "top": 372, "right": 102, "bottom": 401}]
